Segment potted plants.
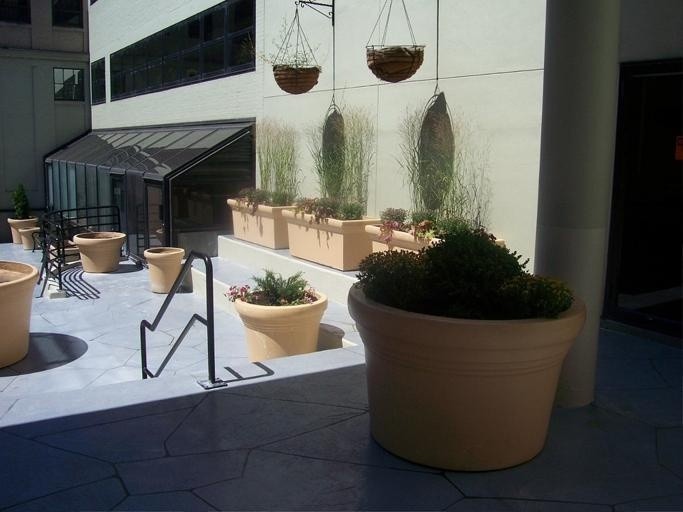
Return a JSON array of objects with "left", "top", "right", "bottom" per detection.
[
  {"left": 282, "top": 102, "right": 378, "bottom": 272},
  {"left": 223, "top": 268, "right": 328, "bottom": 364},
  {"left": 224, "top": 114, "right": 295, "bottom": 250},
  {"left": 241, "top": 11, "right": 323, "bottom": 95},
  {"left": 346, "top": 213, "right": 590, "bottom": 474},
  {"left": 363, "top": 105, "right": 493, "bottom": 258},
  {"left": 7, "top": 180, "right": 40, "bottom": 245}
]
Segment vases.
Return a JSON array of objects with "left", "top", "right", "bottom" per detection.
[
  {"left": 18, "top": 227, "right": 41, "bottom": 250},
  {"left": 71, "top": 231, "right": 127, "bottom": 274},
  {"left": 0, "top": 260, "right": 39, "bottom": 370},
  {"left": 155, "top": 227, "right": 164, "bottom": 245},
  {"left": 143, "top": 246, "right": 185, "bottom": 295},
  {"left": 364, "top": 0, "right": 425, "bottom": 83}
]
